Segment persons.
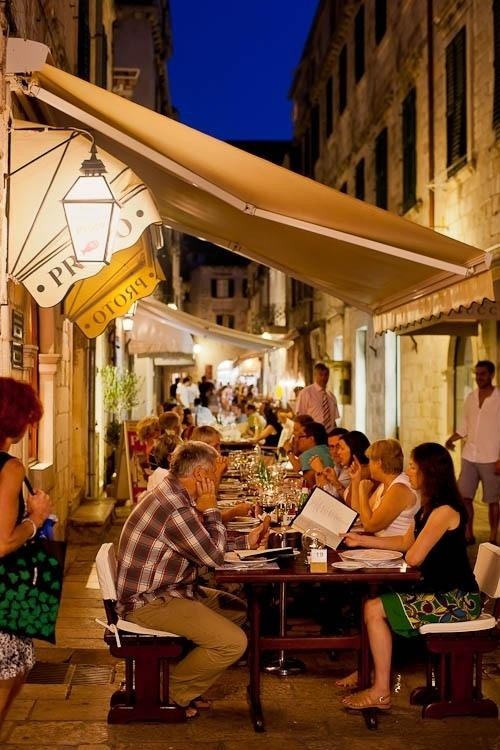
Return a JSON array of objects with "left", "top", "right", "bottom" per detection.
[
  {"left": 114, "top": 442, "right": 271, "bottom": 722},
  {"left": 335, "top": 442, "right": 482, "bottom": 709},
  {"left": 0, "top": 376, "right": 59, "bottom": 727},
  {"left": 446, "top": 360, "right": 500, "bottom": 545},
  {"left": 137, "top": 363, "right": 382, "bottom": 509},
  {"left": 348, "top": 439, "right": 422, "bottom": 537}
]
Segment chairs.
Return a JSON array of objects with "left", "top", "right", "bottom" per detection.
[
  {"left": 410, "top": 540, "right": 500, "bottom": 719},
  {"left": 96, "top": 542, "right": 184, "bottom": 724}
]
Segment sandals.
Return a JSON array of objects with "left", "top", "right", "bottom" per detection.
[
  {"left": 333, "top": 671, "right": 370, "bottom": 689},
  {"left": 172, "top": 700, "right": 200, "bottom": 722},
  {"left": 193, "top": 696, "right": 212, "bottom": 711},
  {"left": 341, "top": 690, "right": 392, "bottom": 712}
]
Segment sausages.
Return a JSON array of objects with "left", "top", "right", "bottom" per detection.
[{"left": 256, "top": 514, "right": 271, "bottom": 545}]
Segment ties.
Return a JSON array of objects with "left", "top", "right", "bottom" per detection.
[{"left": 322, "top": 393, "right": 332, "bottom": 430}]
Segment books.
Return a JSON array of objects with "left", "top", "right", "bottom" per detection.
[{"left": 288, "top": 485, "right": 360, "bottom": 551}]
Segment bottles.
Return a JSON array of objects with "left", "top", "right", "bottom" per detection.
[{"left": 298, "top": 488, "right": 309, "bottom": 513}]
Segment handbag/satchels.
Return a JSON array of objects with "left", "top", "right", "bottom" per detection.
[{"left": 0, "top": 535, "right": 68, "bottom": 646}]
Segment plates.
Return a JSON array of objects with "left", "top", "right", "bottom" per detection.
[
  {"left": 226, "top": 517, "right": 261, "bottom": 527},
  {"left": 217, "top": 500, "right": 247, "bottom": 509},
  {"left": 222, "top": 552, "right": 277, "bottom": 566},
  {"left": 342, "top": 548, "right": 404, "bottom": 564},
  {"left": 332, "top": 561, "right": 363, "bottom": 571}
]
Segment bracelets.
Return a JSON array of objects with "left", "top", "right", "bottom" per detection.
[{"left": 22, "top": 518, "right": 36, "bottom": 539}]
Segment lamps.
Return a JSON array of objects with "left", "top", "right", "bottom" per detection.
[
  {"left": 121, "top": 300, "right": 137, "bottom": 332},
  {"left": 3, "top": 116, "right": 121, "bottom": 266}
]
